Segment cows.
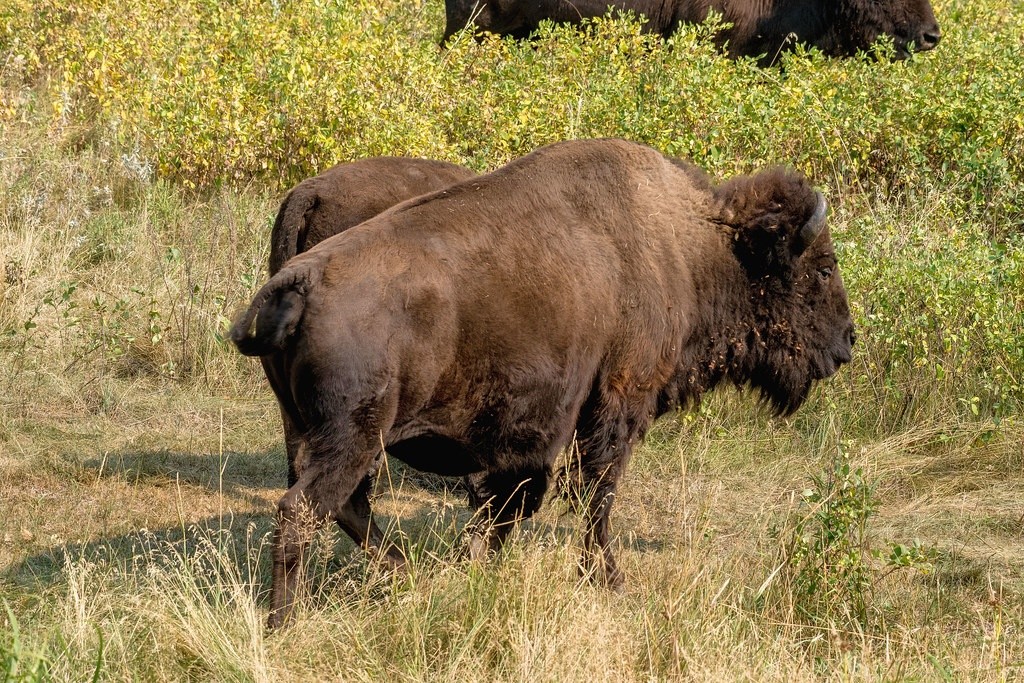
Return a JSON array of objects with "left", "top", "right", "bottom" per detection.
[
  {"left": 229, "top": 133, "right": 861, "bottom": 634},
  {"left": 435, "top": 0, "right": 943, "bottom": 71}
]
